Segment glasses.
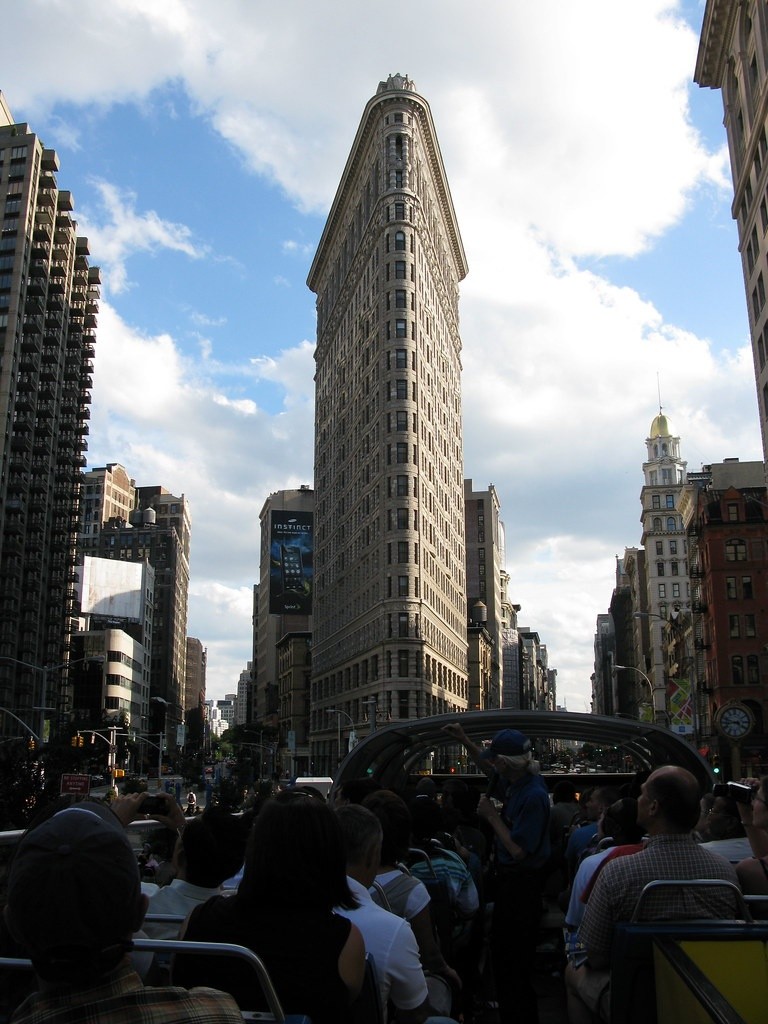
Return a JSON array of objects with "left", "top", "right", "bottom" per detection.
[
  {"left": 176, "top": 823, "right": 183, "bottom": 840},
  {"left": 277, "top": 788, "right": 313, "bottom": 804},
  {"left": 705, "top": 807, "right": 732, "bottom": 819},
  {"left": 750, "top": 792, "right": 767, "bottom": 807}
]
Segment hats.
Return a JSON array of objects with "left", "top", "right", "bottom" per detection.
[
  {"left": 478, "top": 729, "right": 531, "bottom": 758},
  {"left": 6, "top": 802, "right": 143, "bottom": 961}
]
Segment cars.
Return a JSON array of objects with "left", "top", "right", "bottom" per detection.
[
  {"left": 148, "top": 764, "right": 214, "bottom": 781},
  {"left": 551, "top": 760, "right": 604, "bottom": 773}
]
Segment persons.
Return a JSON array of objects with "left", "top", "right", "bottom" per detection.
[
  {"left": 108, "top": 790, "right": 248, "bottom": 962},
  {"left": 549, "top": 765, "right": 768, "bottom": 1024},
  {"left": 186, "top": 792, "right": 196, "bottom": 812},
  {"left": 442, "top": 720, "right": 551, "bottom": 1024},
  {"left": 285, "top": 769, "right": 289, "bottom": 779},
  {"left": 1, "top": 774, "right": 486, "bottom": 1024},
  {"left": 0, "top": 800, "right": 247, "bottom": 1024}
]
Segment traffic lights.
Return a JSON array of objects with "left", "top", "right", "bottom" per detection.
[
  {"left": 71, "top": 736, "right": 76, "bottom": 747},
  {"left": 714, "top": 754, "right": 720, "bottom": 775},
  {"left": 31, "top": 741, "right": 35, "bottom": 750},
  {"left": 80, "top": 736, "right": 84, "bottom": 748},
  {"left": 28, "top": 741, "right": 31, "bottom": 750}
]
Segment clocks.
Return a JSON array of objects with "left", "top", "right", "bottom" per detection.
[{"left": 720, "top": 707, "right": 750, "bottom": 737}]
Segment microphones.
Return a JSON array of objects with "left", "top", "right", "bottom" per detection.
[{"left": 486, "top": 782, "right": 495, "bottom": 798}]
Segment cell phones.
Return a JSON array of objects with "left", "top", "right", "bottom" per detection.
[{"left": 137, "top": 797, "right": 166, "bottom": 816}]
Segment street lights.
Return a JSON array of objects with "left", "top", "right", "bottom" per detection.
[
  {"left": 39, "top": 654, "right": 106, "bottom": 747},
  {"left": 325, "top": 709, "right": 354, "bottom": 733},
  {"left": 613, "top": 664, "right": 653, "bottom": 694}
]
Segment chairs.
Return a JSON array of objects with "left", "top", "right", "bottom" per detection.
[{"left": 0, "top": 830, "right": 768, "bottom": 1024}]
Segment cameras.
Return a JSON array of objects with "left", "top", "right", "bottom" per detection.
[{"left": 712, "top": 779, "right": 759, "bottom": 808}]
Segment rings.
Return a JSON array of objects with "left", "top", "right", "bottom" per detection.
[{"left": 144, "top": 813, "right": 150, "bottom": 819}]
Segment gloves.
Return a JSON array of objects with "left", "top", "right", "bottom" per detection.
[{"left": 437, "top": 965, "right": 462, "bottom": 991}]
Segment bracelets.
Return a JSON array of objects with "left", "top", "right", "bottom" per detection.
[{"left": 740, "top": 819, "right": 753, "bottom": 828}]
